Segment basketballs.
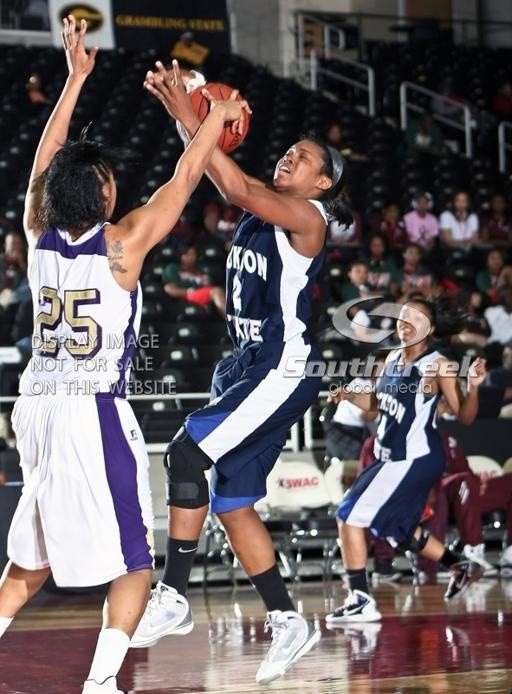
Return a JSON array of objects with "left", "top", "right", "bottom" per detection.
[{"left": 186, "top": 83, "right": 249, "bottom": 154}]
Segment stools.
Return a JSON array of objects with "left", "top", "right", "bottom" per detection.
[{"left": 198, "top": 509, "right": 339, "bottom": 597}]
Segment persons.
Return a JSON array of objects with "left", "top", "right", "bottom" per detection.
[
  {"left": 324, "top": 623, "right": 477, "bottom": 694},
  {"left": 0, "top": 30, "right": 511, "bottom": 586},
  {"left": 0, "top": 15, "right": 252, "bottom": 693},
  {"left": 323, "top": 299, "right": 488, "bottom": 625},
  {"left": 127, "top": 57, "right": 349, "bottom": 686}
]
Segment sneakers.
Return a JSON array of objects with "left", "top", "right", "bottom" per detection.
[
  {"left": 255, "top": 609, "right": 322, "bottom": 686},
  {"left": 461, "top": 543, "right": 498, "bottom": 576},
  {"left": 325, "top": 589, "right": 383, "bottom": 623},
  {"left": 444, "top": 554, "right": 484, "bottom": 599},
  {"left": 129, "top": 580, "right": 195, "bottom": 649},
  {"left": 495, "top": 544, "right": 512, "bottom": 577},
  {"left": 370, "top": 571, "right": 401, "bottom": 582}
]
